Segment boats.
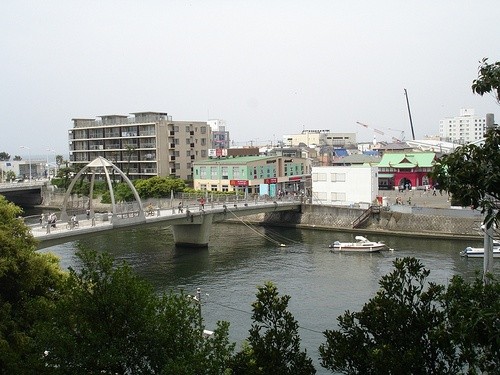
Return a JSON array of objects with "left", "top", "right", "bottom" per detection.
[
  {"left": 328, "top": 236, "right": 386, "bottom": 252},
  {"left": 459, "top": 239, "right": 500, "bottom": 258}
]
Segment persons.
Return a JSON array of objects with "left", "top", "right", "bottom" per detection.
[
  {"left": 178, "top": 202, "right": 183, "bottom": 213},
  {"left": 39, "top": 213, "right": 45, "bottom": 228},
  {"left": 147, "top": 202, "right": 154, "bottom": 215},
  {"left": 433, "top": 187, "right": 436, "bottom": 196},
  {"left": 376, "top": 196, "right": 382, "bottom": 204},
  {"left": 71, "top": 214, "right": 78, "bottom": 226},
  {"left": 278, "top": 188, "right": 312, "bottom": 199},
  {"left": 396, "top": 196, "right": 402, "bottom": 205},
  {"left": 200, "top": 196, "right": 205, "bottom": 209},
  {"left": 47, "top": 213, "right": 57, "bottom": 228}
]
